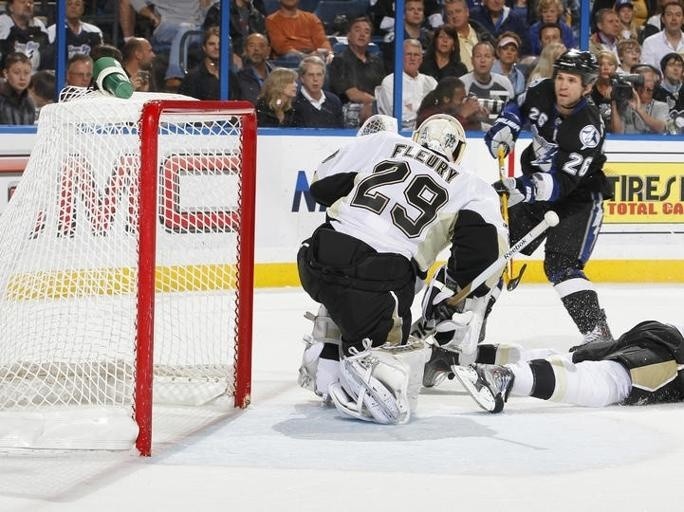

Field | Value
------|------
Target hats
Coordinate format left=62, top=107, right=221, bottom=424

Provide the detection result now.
left=615, top=0, right=633, bottom=10
left=497, top=37, right=519, bottom=48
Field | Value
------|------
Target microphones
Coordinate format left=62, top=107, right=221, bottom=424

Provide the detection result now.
left=647, top=88, right=657, bottom=91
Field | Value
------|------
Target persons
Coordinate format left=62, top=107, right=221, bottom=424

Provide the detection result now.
left=449, top=319, right=684, bottom=414
left=1, top=0, right=684, bottom=137
left=423, top=48, right=617, bottom=387
left=675, top=412, right=678, bottom=415
left=494, top=422, right=499, bottom=424
left=295, top=112, right=510, bottom=426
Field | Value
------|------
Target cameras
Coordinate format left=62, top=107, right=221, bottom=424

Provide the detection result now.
left=138, top=71, right=152, bottom=81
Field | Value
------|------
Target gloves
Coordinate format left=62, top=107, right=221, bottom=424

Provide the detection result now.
left=492, top=176, right=537, bottom=209
left=486, top=111, right=521, bottom=159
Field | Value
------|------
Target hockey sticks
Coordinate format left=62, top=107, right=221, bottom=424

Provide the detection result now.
left=496, top=146, right=526, bottom=291
left=412, top=209, right=562, bottom=340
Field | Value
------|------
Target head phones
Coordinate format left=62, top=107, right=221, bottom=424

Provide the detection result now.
left=630, top=64, right=661, bottom=89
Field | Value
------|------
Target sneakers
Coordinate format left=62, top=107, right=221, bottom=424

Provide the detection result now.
left=471, top=362, right=513, bottom=402
left=568, top=321, right=616, bottom=363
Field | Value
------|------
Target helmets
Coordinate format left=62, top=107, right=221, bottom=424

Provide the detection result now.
left=411, top=113, right=467, bottom=166
left=551, top=50, right=600, bottom=86
